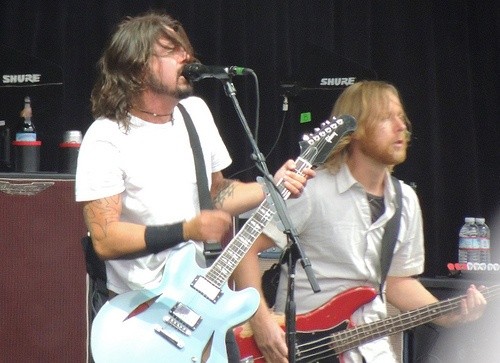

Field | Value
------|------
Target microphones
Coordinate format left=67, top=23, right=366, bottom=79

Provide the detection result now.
left=182, top=62, right=255, bottom=81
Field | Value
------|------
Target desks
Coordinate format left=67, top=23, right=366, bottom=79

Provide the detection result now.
left=404, top=274, right=500, bottom=363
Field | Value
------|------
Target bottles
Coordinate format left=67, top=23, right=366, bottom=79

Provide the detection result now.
left=16, top=96, right=36, bottom=142
left=475, top=218, right=490, bottom=272
left=459, top=217, right=479, bottom=274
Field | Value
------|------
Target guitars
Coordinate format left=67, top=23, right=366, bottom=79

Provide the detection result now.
left=89, top=113, right=358, bottom=363
left=232, top=280, right=500, bottom=363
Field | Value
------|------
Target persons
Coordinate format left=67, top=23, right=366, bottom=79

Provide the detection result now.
left=234, top=81, right=487, bottom=363
left=74, top=15, right=315, bottom=363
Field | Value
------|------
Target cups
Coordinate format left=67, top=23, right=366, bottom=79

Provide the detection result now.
left=64, top=130, right=82, bottom=143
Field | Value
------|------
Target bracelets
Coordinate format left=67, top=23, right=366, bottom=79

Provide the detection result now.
left=145, top=219, right=186, bottom=254
left=141, top=109, right=169, bottom=117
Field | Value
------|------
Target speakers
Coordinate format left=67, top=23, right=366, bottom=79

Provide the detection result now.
left=386, top=279, right=500, bottom=363
left=0, top=171, right=94, bottom=362
